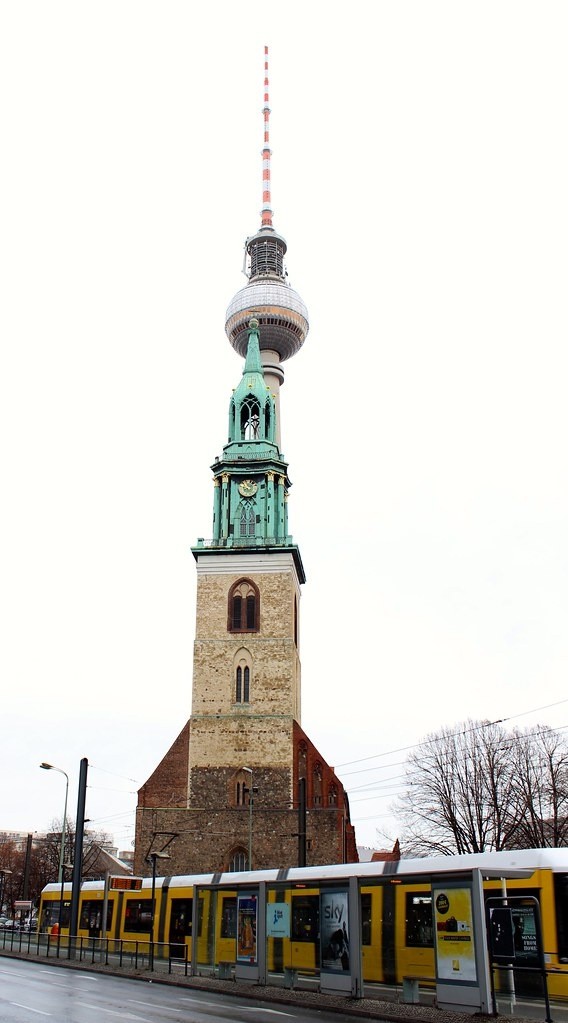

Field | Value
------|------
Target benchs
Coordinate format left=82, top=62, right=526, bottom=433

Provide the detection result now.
left=219, top=962, right=236, bottom=979
left=284, top=965, right=320, bottom=988
left=402, top=975, right=436, bottom=1004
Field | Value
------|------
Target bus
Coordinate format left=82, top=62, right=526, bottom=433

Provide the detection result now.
left=34, top=848, right=568, bottom=1006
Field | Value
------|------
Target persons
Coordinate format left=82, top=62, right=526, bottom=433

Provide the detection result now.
left=494, top=917, right=524, bottom=995
left=81, top=911, right=101, bottom=936
left=364, top=907, right=424, bottom=974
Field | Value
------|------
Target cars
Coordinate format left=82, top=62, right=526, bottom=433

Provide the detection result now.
left=0, top=916, right=38, bottom=932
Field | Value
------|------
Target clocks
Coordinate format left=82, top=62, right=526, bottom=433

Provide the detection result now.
left=238, top=479, right=257, bottom=497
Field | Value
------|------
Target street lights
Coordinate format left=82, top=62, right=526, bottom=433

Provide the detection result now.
left=0, top=869, right=13, bottom=923
left=147, top=852, right=172, bottom=971
left=242, top=766, right=253, bottom=870
left=57, top=862, right=73, bottom=959
left=39, top=763, right=69, bottom=883
